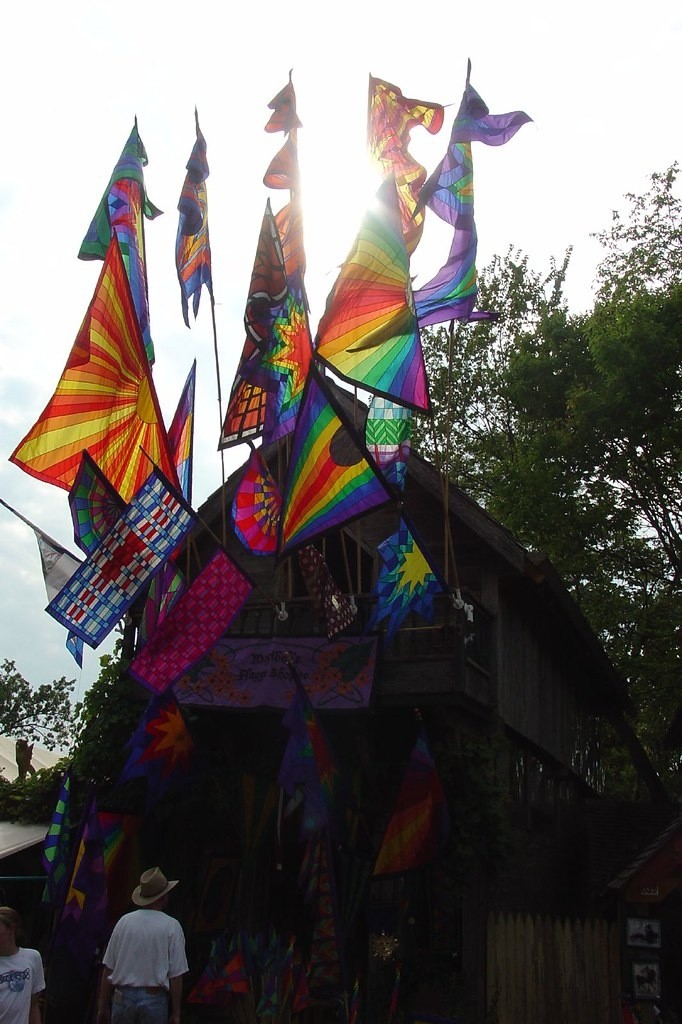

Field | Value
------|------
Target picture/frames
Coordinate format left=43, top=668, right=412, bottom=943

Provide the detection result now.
left=626, top=917, right=662, bottom=949
left=631, top=960, right=662, bottom=1001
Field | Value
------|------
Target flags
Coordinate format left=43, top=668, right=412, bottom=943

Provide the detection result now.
left=45, top=467, right=198, bottom=650
left=125, top=544, right=257, bottom=694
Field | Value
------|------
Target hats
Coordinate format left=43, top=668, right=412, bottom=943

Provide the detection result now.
left=131, top=867, right=180, bottom=906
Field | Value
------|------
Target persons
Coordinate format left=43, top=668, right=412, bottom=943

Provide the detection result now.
left=96, top=866, right=189, bottom=1024
left=0, top=906, right=46, bottom=1024
